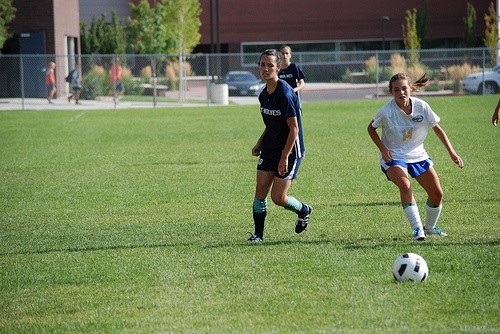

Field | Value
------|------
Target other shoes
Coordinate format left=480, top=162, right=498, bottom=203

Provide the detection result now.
left=75, top=102, right=81, bottom=104
left=68, top=96, right=71, bottom=102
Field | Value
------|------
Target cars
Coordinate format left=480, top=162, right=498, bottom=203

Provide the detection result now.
left=461, top=63, right=500, bottom=95
left=226, top=71, right=261, bottom=96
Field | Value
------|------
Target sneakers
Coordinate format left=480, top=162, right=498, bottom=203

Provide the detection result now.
left=246, top=232, right=263, bottom=243
left=412, top=227, right=426, bottom=241
left=423, top=226, right=447, bottom=237
left=295, top=204, right=312, bottom=233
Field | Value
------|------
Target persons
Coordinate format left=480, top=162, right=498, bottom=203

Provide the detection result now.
left=278, top=43, right=305, bottom=159
left=367, top=72, right=464, bottom=241
left=492, top=99, right=500, bottom=126
left=42, top=61, right=124, bottom=105
left=243, top=48, right=313, bottom=243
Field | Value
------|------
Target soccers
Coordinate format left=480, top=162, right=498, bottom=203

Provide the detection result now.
left=393, top=253, right=428, bottom=283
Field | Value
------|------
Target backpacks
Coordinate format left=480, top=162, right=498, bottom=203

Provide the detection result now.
left=65, top=69, right=79, bottom=82
